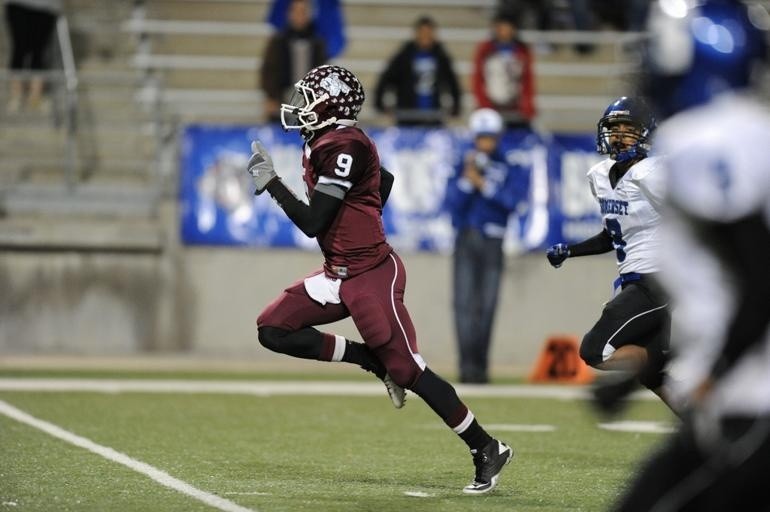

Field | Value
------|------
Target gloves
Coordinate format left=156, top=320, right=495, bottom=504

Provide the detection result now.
left=547, top=243, right=570, bottom=268
left=247, top=141, right=279, bottom=195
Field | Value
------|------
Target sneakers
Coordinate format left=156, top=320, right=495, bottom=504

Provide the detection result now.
left=463, top=438, right=514, bottom=493
left=384, top=373, right=406, bottom=408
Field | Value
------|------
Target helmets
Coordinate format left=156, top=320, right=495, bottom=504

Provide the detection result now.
left=596, top=96, right=658, bottom=161
left=280, top=65, right=365, bottom=133
left=469, top=108, right=500, bottom=135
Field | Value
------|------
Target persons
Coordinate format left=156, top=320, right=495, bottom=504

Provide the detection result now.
left=471, top=10, right=534, bottom=121
left=442, top=107, right=531, bottom=385
left=268, top=0, right=347, bottom=60
left=545, top=96, right=711, bottom=428
left=247, top=64, right=513, bottom=495
left=375, top=19, right=456, bottom=134
left=613, top=0, right=769, bottom=512
